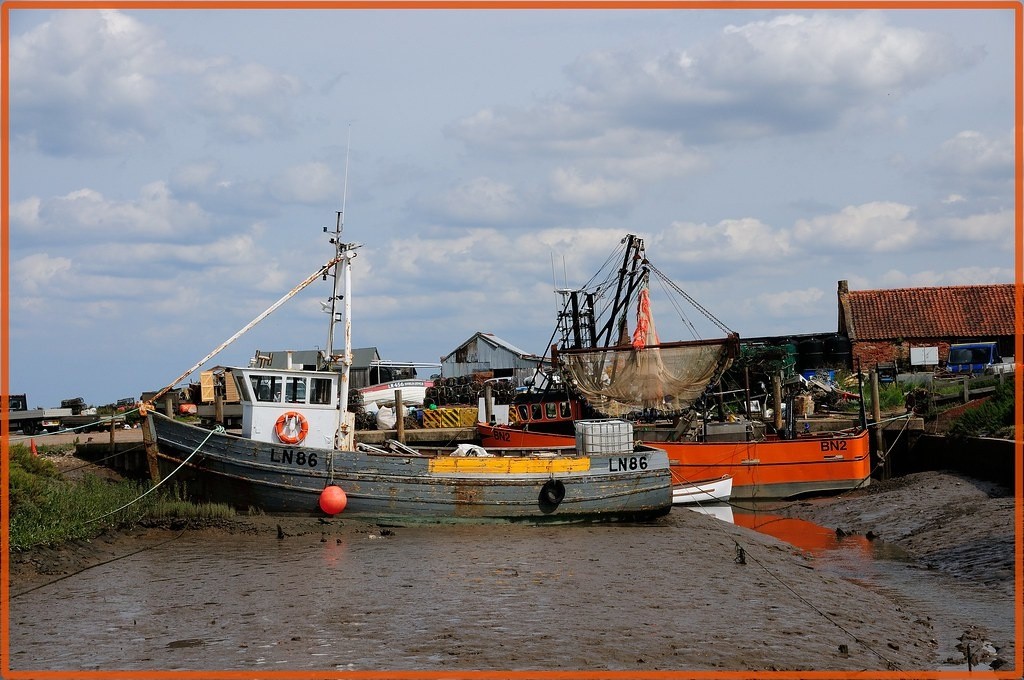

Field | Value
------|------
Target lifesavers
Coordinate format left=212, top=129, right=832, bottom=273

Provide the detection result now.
left=543, top=480, right=566, bottom=502
left=271, top=410, right=311, bottom=447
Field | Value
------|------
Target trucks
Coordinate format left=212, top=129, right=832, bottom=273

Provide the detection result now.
left=9, top=393, right=73, bottom=436
left=37, top=407, right=127, bottom=433
left=945, top=342, right=1004, bottom=379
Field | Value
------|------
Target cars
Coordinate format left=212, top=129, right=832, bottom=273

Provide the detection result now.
left=123, top=424, right=133, bottom=430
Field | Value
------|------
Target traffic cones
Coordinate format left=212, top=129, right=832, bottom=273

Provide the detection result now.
left=29, top=438, right=39, bottom=459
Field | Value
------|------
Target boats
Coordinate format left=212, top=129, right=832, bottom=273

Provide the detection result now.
left=335, top=378, right=435, bottom=409
left=475, top=230, right=871, bottom=500
left=671, top=476, right=735, bottom=505
left=142, top=210, right=675, bottom=523
